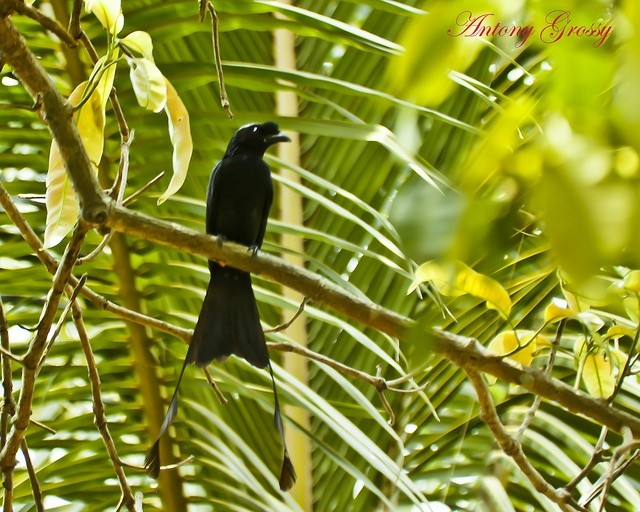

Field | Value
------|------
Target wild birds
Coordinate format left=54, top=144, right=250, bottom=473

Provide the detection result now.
left=144, top=120, right=297, bottom=492
